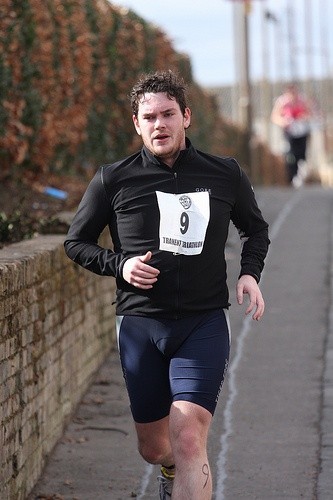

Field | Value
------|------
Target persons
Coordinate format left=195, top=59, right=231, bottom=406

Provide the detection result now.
left=274, top=78, right=313, bottom=189
left=63, top=70, right=272, bottom=500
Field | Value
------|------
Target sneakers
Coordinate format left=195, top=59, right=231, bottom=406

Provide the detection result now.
left=157, top=467, right=175, bottom=500
